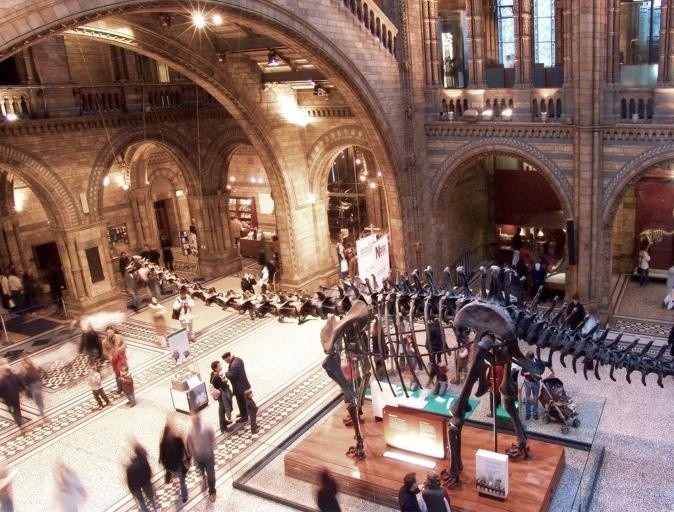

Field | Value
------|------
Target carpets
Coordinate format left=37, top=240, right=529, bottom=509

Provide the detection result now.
left=0, top=311, right=63, bottom=340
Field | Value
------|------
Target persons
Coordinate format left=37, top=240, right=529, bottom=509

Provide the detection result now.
left=421, top=470, right=451, bottom=512
left=317, top=470, right=341, bottom=512
left=335, top=233, right=358, bottom=278
left=662, top=265, right=674, bottom=311
left=511, top=227, right=522, bottom=268
left=398, top=472, right=428, bottom=512
left=566, top=295, right=584, bottom=340
left=344, top=315, right=576, bottom=421
left=229, top=215, right=244, bottom=246
left=638, top=243, right=651, bottom=286
left=581, top=304, right=599, bottom=340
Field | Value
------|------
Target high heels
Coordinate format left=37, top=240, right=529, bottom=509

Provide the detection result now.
left=221, top=428, right=233, bottom=434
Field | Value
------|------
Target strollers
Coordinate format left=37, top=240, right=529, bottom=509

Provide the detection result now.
left=536, top=376, right=581, bottom=435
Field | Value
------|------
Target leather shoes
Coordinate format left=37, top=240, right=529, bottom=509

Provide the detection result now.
left=236, top=415, right=248, bottom=422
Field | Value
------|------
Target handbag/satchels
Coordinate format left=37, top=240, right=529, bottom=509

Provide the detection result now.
left=171, top=299, right=182, bottom=320
left=209, top=385, right=220, bottom=400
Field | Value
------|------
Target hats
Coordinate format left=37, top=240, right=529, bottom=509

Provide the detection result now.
left=427, top=469, right=440, bottom=481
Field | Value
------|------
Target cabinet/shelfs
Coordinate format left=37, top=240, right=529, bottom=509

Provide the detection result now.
left=226, top=194, right=258, bottom=234
left=240, top=236, right=274, bottom=260
